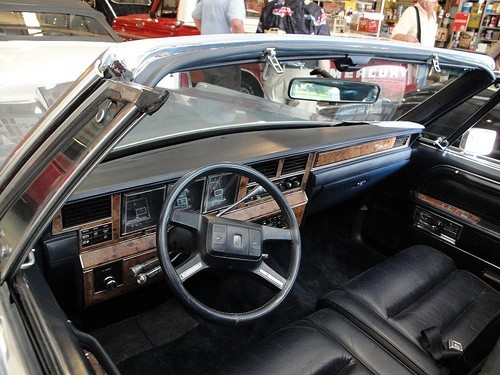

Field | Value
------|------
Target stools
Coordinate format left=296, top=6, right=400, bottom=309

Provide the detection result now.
left=224, top=244, right=500, bottom=375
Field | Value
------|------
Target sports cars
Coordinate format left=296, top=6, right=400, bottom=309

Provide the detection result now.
left=0, top=26, right=500, bottom=375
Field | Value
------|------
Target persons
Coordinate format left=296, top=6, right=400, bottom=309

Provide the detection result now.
left=331, top=10, right=353, bottom=25
left=391, top=0, right=439, bottom=87
left=254, top=0, right=331, bottom=113
left=192, top=0, right=246, bottom=90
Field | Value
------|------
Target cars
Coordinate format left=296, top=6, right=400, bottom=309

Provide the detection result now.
left=0, top=0, right=417, bottom=107
